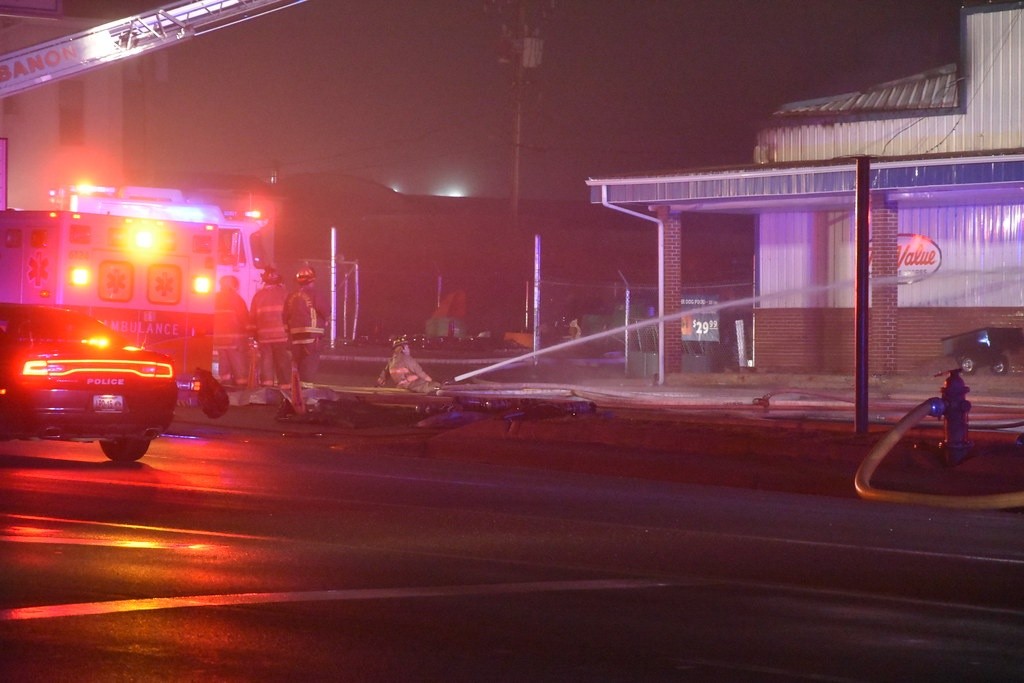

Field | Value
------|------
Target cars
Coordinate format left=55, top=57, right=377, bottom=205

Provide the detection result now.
left=0, top=301, right=178, bottom=462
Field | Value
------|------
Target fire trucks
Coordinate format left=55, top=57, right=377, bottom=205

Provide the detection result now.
left=72, top=198, right=268, bottom=314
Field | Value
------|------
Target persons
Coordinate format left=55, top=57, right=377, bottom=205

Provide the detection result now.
left=214, top=275, right=254, bottom=387
left=245, top=269, right=293, bottom=386
left=283, top=265, right=328, bottom=383
left=376, top=337, right=443, bottom=393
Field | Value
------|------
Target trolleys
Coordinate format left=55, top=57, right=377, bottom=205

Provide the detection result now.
left=941, top=326, right=1024, bottom=376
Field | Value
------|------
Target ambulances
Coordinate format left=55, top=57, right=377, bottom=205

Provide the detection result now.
left=0, top=208, right=219, bottom=412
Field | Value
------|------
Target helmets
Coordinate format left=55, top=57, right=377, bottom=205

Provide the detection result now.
left=391, top=337, right=408, bottom=351
left=293, top=266, right=316, bottom=286
